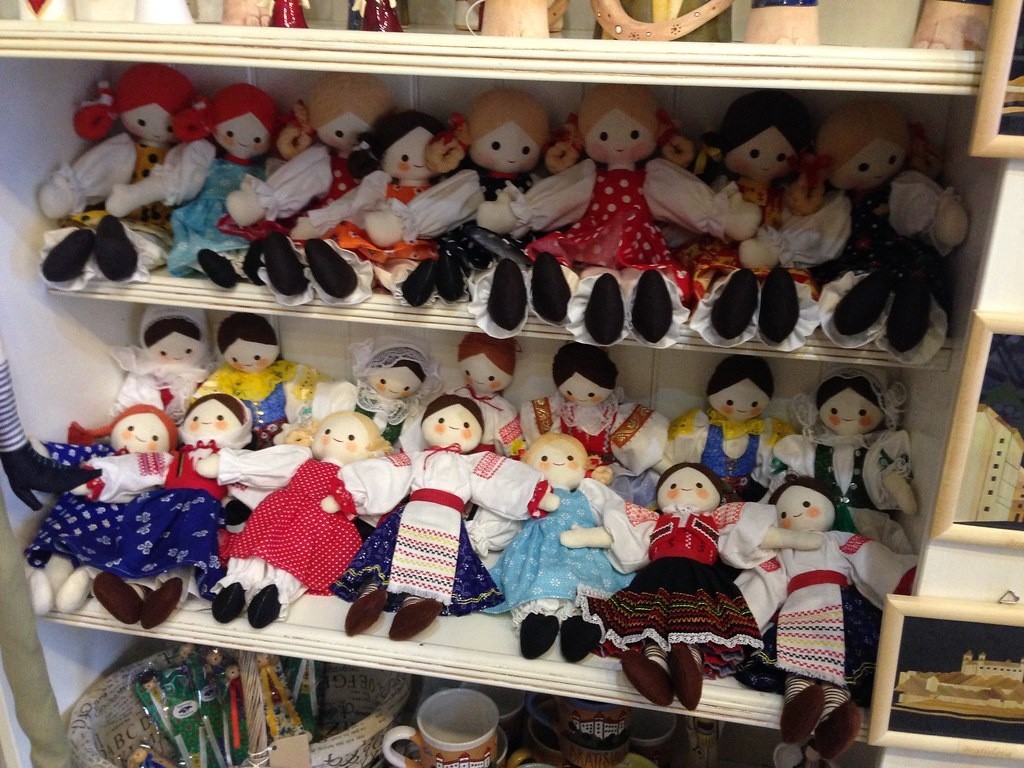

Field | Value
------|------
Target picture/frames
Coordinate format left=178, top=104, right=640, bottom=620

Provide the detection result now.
left=864, top=590, right=1024, bottom=758
left=968, top=0, right=1024, bottom=158
left=927, top=309, right=1024, bottom=550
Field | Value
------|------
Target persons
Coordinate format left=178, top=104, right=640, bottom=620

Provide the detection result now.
left=40, top=64, right=969, bottom=368
left=259, top=0, right=311, bottom=28
left=24, top=302, right=918, bottom=758
left=0, top=340, right=101, bottom=511
left=352, top=0, right=403, bottom=32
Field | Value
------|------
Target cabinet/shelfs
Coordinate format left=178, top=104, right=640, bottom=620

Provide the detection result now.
left=0, top=1, right=984, bottom=768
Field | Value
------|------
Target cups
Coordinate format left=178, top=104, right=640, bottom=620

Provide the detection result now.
left=382, top=676, right=680, bottom=768
